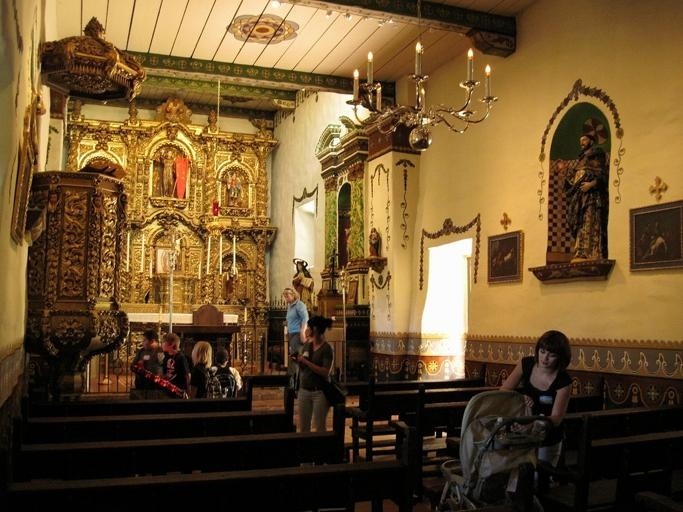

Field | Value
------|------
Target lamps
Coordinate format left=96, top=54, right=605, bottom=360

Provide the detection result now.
left=346, top=1, right=498, bottom=150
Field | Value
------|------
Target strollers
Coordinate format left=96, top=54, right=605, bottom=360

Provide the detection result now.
left=429, top=387, right=561, bottom=509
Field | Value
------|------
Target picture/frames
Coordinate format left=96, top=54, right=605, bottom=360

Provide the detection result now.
left=630, top=199, right=682, bottom=272
left=488, top=230, right=523, bottom=284
left=10, top=138, right=34, bottom=245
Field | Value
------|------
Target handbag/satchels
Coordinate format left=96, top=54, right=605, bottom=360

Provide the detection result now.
left=321, top=379, right=348, bottom=406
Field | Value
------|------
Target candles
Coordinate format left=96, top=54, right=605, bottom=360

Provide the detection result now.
left=207, top=233, right=236, bottom=276
left=127, top=232, right=153, bottom=278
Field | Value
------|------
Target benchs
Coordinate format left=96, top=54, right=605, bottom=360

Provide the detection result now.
left=5, top=396, right=400, bottom=510
left=336, top=379, right=502, bottom=510
left=536, top=394, right=681, bottom=512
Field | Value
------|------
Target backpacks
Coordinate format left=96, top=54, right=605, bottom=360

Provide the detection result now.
left=194, top=366, right=238, bottom=399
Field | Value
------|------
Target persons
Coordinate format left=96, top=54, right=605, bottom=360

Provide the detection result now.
left=566, top=118, right=611, bottom=261
left=130, top=330, right=164, bottom=389
left=187, top=340, right=213, bottom=399
left=211, top=347, right=243, bottom=399
left=499, top=329, right=572, bottom=480
left=160, top=148, right=177, bottom=197
left=226, top=169, right=243, bottom=207
left=292, top=261, right=315, bottom=313
left=283, top=287, right=310, bottom=389
left=158, top=331, right=187, bottom=399
left=294, top=314, right=334, bottom=433
